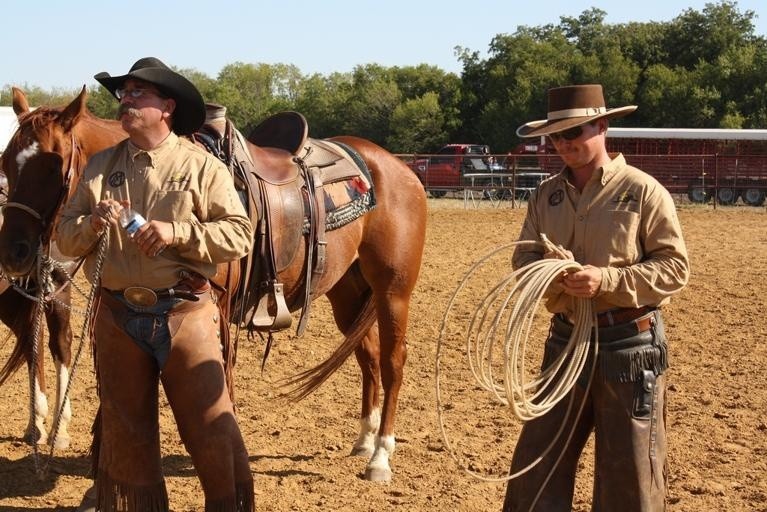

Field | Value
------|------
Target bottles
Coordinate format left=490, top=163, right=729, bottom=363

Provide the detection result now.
left=117, top=205, right=165, bottom=258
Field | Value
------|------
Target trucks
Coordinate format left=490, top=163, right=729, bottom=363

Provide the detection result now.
left=502, top=133, right=765, bottom=209
left=408, top=143, right=539, bottom=201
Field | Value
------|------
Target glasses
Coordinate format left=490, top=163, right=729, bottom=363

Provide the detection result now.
left=550, top=126, right=582, bottom=141
left=115, top=87, right=145, bottom=99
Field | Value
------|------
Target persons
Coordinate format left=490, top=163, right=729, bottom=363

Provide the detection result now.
left=500, top=81, right=693, bottom=510
left=48, top=55, right=257, bottom=510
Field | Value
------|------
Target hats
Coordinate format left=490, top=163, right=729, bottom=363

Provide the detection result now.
left=94, top=57, right=206, bottom=136
left=516, top=83, right=638, bottom=137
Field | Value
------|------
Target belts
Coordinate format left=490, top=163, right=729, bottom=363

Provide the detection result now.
left=556, top=306, right=656, bottom=336
left=103, top=289, right=201, bottom=303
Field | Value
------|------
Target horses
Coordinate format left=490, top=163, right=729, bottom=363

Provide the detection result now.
left=0, top=86, right=431, bottom=482
left=0, top=169, right=79, bottom=453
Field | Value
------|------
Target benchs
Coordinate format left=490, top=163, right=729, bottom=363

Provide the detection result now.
left=462, top=173, right=550, bottom=208
